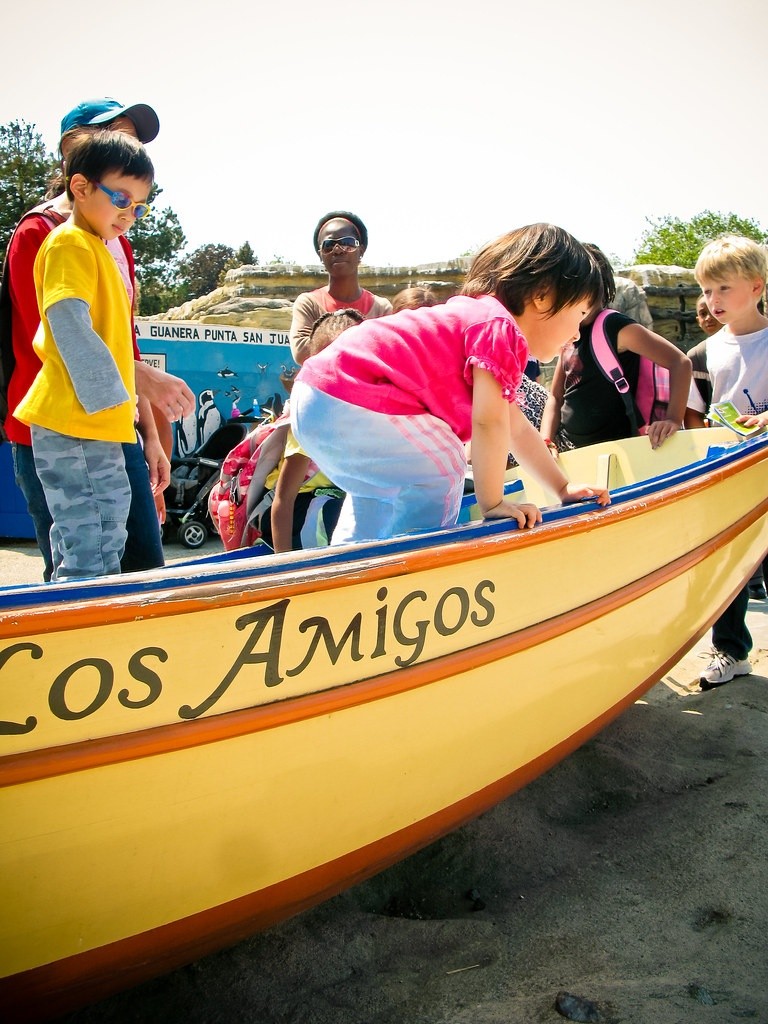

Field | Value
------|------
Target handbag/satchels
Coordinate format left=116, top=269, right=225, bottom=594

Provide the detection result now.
left=589, top=308, right=683, bottom=436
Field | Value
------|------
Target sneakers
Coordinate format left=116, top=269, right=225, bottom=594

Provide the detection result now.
left=697, top=646, right=753, bottom=690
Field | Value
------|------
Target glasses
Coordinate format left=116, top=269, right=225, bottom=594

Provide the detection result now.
left=66, top=172, right=151, bottom=219
left=318, top=236, right=359, bottom=256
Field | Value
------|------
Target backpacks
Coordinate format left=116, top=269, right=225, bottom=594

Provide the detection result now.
left=208, top=418, right=292, bottom=552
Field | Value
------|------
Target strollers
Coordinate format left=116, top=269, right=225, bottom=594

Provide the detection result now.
left=161, top=407, right=275, bottom=549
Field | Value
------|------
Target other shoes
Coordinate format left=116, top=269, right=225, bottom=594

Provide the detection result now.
left=747, top=584, right=767, bottom=599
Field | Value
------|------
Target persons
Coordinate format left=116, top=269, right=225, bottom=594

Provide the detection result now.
left=687, top=236, right=768, bottom=688
left=3, top=96, right=196, bottom=582
left=254, top=211, right=692, bottom=553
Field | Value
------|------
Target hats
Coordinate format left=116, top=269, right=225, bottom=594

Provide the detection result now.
left=313, top=211, right=368, bottom=253
left=61, top=98, right=160, bottom=144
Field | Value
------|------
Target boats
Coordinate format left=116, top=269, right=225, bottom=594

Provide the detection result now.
left=0, top=428, right=767, bottom=1024
